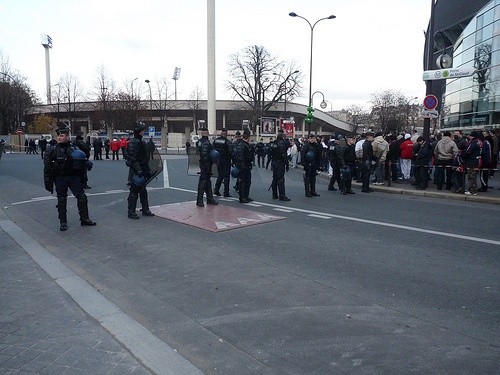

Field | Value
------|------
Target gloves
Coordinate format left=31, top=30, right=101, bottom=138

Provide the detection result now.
left=135, top=169, right=143, bottom=177
left=286, top=166, right=289, bottom=172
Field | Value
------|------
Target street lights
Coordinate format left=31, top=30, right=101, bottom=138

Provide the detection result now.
left=272, top=71, right=301, bottom=142
left=399, top=97, right=418, bottom=129
left=288, top=12, right=336, bottom=140
left=144, top=80, right=152, bottom=142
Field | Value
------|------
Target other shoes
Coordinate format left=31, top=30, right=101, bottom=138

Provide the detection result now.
left=214, top=191, right=221, bottom=196
left=341, top=189, right=355, bottom=195
left=465, top=191, right=477, bottom=196
left=196, top=202, right=204, bottom=207
left=306, top=191, right=320, bottom=198
left=233, top=186, right=238, bottom=191
left=128, top=214, right=139, bottom=219
left=273, top=194, right=291, bottom=201
left=361, top=188, right=374, bottom=193
left=373, top=182, right=384, bottom=186
left=207, top=200, right=218, bottom=205
left=328, top=187, right=337, bottom=191
left=60, top=223, right=68, bottom=231
left=224, top=195, right=232, bottom=197
left=240, top=198, right=254, bottom=203
left=142, top=211, right=155, bottom=216
left=81, top=220, right=96, bottom=226
left=478, top=187, right=488, bottom=192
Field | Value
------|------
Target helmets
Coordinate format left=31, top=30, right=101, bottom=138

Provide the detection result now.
left=210, top=150, right=220, bottom=162
left=71, top=150, right=86, bottom=160
left=231, top=167, right=239, bottom=178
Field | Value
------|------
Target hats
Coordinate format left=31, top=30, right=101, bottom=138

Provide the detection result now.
left=132, top=124, right=147, bottom=133
left=56, top=128, right=71, bottom=135
left=405, top=134, right=411, bottom=140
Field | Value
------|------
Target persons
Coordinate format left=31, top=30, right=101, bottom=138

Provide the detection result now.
left=186, top=140, right=191, bottom=155
left=24, top=136, right=57, bottom=159
left=0, top=139, right=6, bottom=159
left=124, top=123, right=155, bottom=219
left=75, top=132, right=129, bottom=161
left=42, top=127, right=97, bottom=231
left=195, top=124, right=500, bottom=207
